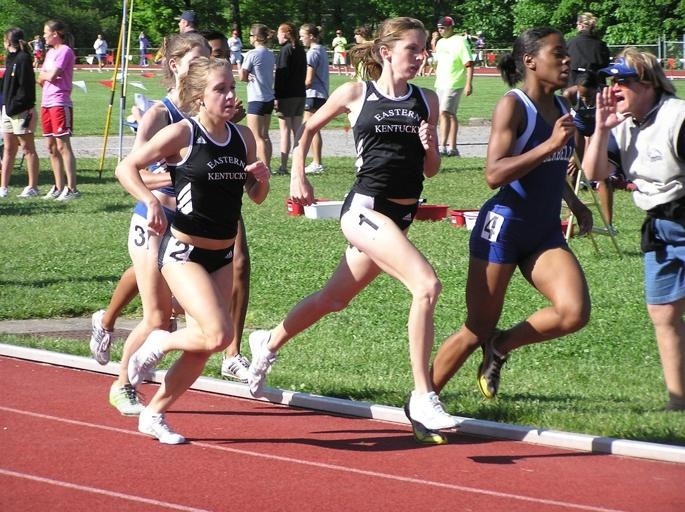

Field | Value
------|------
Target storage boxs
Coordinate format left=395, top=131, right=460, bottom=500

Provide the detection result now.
left=415, top=204, right=482, bottom=232
left=561, top=219, right=577, bottom=238
left=303, top=200, right=345, bottom=219
left=285, top=197, right=328, bottom=216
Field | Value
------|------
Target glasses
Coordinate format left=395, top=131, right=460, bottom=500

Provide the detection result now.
left=610, top=78, right=639, bottom=85
left=249, top=34, right=254, bottom=37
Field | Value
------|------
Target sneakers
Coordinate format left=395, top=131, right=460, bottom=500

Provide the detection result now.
left=404, top=403, right=448, bottom=444
left=220, top=353, right=251, bottom=380
left=55, top=186, right=81, bottom=201
left=304, top=163, right=324, bottom=174
left=108, top=380, right=146, bottom=417
left=90, top=309, right=114, bottom=365
left=128, top=329, right=170, bottom=387
left=447, top=148, right=459, bottom=157
left=16, top=186, right=39, bottom=197
left=247, top=330, right=276, bottom=397
left=409, top=395, right=461, bottom=430
left=0, top=186, right=8, bottom=196
left=273, top=167, right=288, bottom=175
left=138, top=409, right=187, bottom=444
left=477, top=328, right=511, bottom=399
left=40, top=185, right=63, bottom=199
left=438, top=146, right=447, bottom=154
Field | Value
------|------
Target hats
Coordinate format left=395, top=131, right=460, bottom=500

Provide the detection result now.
left=174, top=10, right=195, bottom=24
left=437, top=16, right=455, bottom=28
left=598, top=57, right=658, bottom=80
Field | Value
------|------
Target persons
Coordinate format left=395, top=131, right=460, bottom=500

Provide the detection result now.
left=404, top=25, right=593, bottom=447
left=582, top=45, right=684, bottom=414
left=247, top=14, right=460, bottom=432
left=89, top=31, right=252, bottom=383
left=109, top=34, right=212, bottom=416
left=88, top=57, right=271, bottom=447
left=1, top=10, right=684, bottom=203
